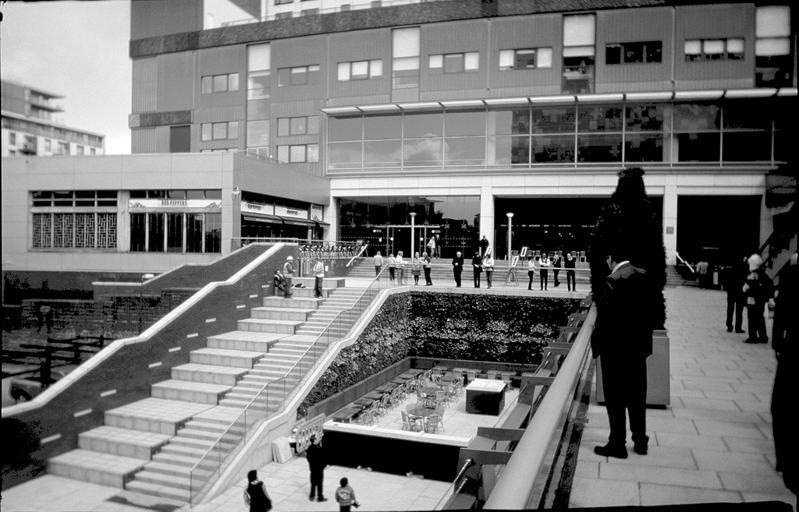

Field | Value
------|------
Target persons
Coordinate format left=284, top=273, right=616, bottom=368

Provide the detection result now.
left=412, top=251, right=423, bottom=286
left=590, top=240, right=656, bottom=460
left=451, top=251, right=464, bottom=288
left=372, top=250, right=383, bottom=280
left=427, top=232, right=441, bottom=258
left=539, top=252, right=550, bottom=291
left=420, top=252, right=433, bottom=286
left=471, top=234, right=495, bottom=289
left=551, top=254, right=561, bottom=287
left=564, top=252, right=576, bottom=291
left=243, top=469, right=273, bottom=512
left=312, top=257, right=325, bottom=299
left=387, top=253, right=396, bottom=280
left=283, top=256, right=296, bottom=299
left=394, top=250, right=408, bottom=287
left=305, top=435, right=329, bottom=502
left=335, top=476, right=361, bottom=512
left=273, top=269, right=294, bottom=292
left=697, top=252, right=799, bottom=352
left=527, top=256, right=536, bottom=290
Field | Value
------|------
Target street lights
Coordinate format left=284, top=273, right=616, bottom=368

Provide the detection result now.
left=505, top=211, right=515, bottom=267
left=36, top=303, right=55, bottom=392
left=409, top=212, right=418, bottom=263
left=139, top=272, right=157, bottom=335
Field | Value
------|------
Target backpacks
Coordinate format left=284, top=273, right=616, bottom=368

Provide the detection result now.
left=752, top=272, right=776, bottom=304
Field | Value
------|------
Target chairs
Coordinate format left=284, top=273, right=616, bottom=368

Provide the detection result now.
left=330, top=365, right=521, bottom=434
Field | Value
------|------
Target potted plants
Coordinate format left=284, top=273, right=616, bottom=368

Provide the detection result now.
left=593, top=166, right=671, bottom=409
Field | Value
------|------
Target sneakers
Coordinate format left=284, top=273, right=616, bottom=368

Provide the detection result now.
left=727, top=327, right=767, bottom=344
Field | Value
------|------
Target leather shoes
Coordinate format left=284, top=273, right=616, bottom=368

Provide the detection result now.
left=527, top=282, right=576, bottom=292
left=317, top=498, right=327, bottom=501
left=633, top=444, right=648, bottom=455
left=398, top=282, right=492, bottom=289
left=594, top=443, right=628, bottom=459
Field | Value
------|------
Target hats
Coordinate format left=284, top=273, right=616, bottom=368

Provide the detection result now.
left=748, top=255, right=764, bottom=270
left=287, top=255, right=295, bottom=260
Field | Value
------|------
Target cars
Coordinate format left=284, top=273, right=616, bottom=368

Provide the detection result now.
left=7, top=364, right=81, bottom=407
